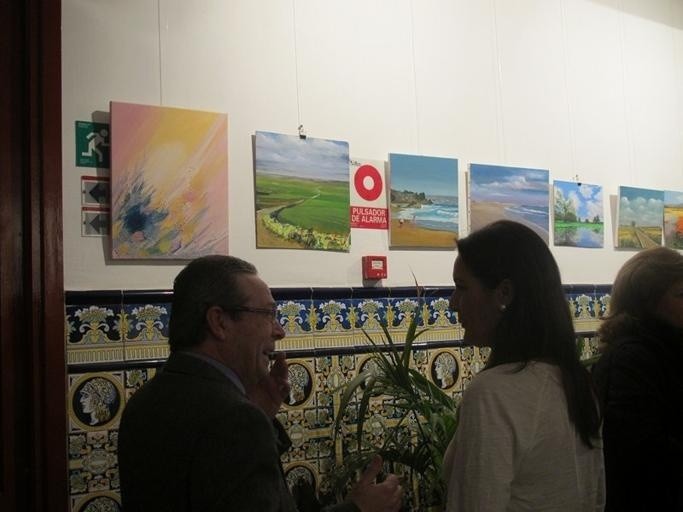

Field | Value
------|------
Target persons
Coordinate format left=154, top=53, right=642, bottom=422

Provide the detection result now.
left=118, top=255, right=404, bottom=512
left=442, top=221, right=607, bottom=512
left=591, top=248, right=683, bottom=511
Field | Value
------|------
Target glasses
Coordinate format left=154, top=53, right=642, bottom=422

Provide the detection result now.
left=224, top=304, right=276, bottom=323
